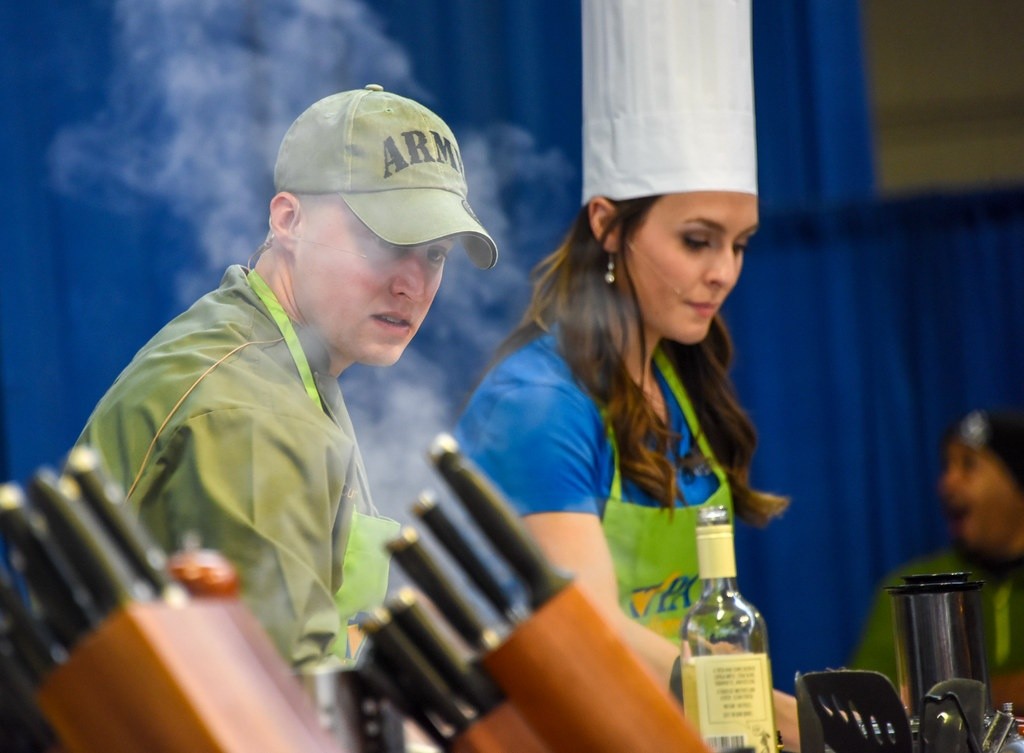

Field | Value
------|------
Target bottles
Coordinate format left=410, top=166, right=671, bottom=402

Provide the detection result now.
left=680, top=505, right=778, bottom=752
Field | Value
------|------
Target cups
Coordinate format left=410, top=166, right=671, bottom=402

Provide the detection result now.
left=887, top=571, right=992, bottom=726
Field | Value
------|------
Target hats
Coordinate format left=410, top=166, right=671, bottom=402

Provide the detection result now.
left=946, top=409, right=1024, bottom=488
left=580, top=0, right=757, bottom=208
left=274, top=85, right=499, bottom=268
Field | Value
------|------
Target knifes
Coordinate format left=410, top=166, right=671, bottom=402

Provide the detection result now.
left=0, top=443, right=190, bottom=696
left=357, top=434, right=574, bottom=751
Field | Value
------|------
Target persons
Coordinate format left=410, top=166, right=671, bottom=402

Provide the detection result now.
left=30, top=84, right=498, bottom=753
left=454, top=0, right=801, bottom=753
left=848, top=408, right=1024, bottom=723
